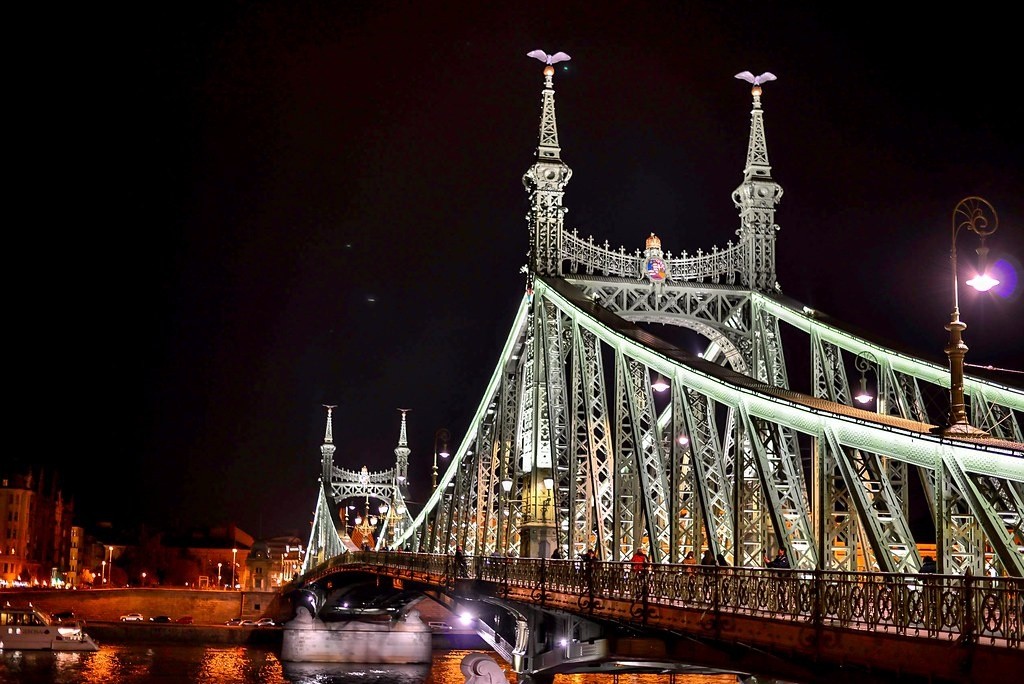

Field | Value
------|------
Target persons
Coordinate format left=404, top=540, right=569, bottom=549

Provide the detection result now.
left=455, top=545, right=469, bottom=578
left=717, top=554, right=731, bottom=598
left=629, top=549, right=649, bottom=573
left=490, top=549, right=500, bottom=557
left=551, top=549, right=561, bottom=559
left=360, top=543, right=425, bottom=563
left=917, top=556, right=936, bottom=592
left=681, top=551, right=697, bottom=591
left=577, top=549, right=599, bottom=560
left=506, top=550, right=514, bottom=558
left=763, top=546, right=791, bottom=578
left=701, top=550, right=715, bottom=573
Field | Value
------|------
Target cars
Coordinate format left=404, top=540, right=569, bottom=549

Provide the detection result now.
left=55, top=612, right=75, bottom=619
left=148, top=616, right=171, bottom=623
left=175, top=616, right=194, bottom=624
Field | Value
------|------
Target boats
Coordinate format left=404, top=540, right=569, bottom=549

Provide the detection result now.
left=0, top=607, right=99, bottom=651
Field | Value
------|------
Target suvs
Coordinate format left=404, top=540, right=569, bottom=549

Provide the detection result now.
left=120, top=613, right=143, bottom=622
left=224, top=618, right=285, bottom=627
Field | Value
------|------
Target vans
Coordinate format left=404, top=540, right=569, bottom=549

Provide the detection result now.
left=428, top=622, right=452, bottom=631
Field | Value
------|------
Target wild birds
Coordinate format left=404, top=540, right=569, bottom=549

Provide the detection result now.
left=734, top=70, right=778, bottom=85
left=527, top=49, right=572, bottom=66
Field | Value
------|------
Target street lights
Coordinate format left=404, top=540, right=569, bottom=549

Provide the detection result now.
left=855, top=351, right=881, bottom=415
left=939, top=197, right=1000, bottom=439
left=108, top=546, right=113, bottom=584
left=232, top=547, right=237, bottom=591
left=218, top=563, right=222, bottom=589
left=102, top=560, right=106, bottom=585
left=430, top=428, right=452, bottom=496
